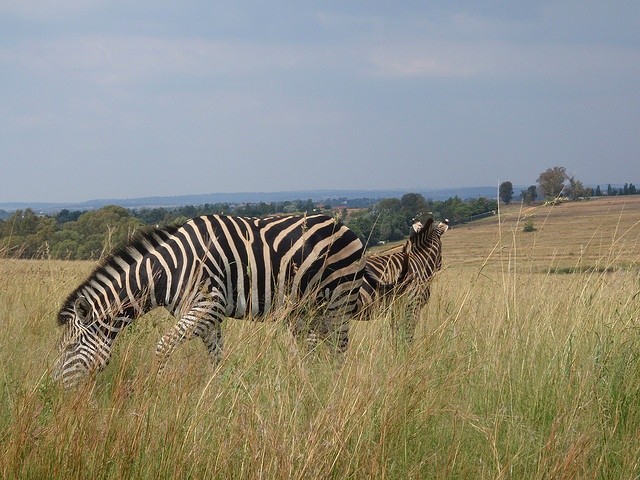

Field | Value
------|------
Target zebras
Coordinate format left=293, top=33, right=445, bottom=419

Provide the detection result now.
left=56, top=213, right=366, bottom=396
left=351, top=218, right=451, bottom=345
